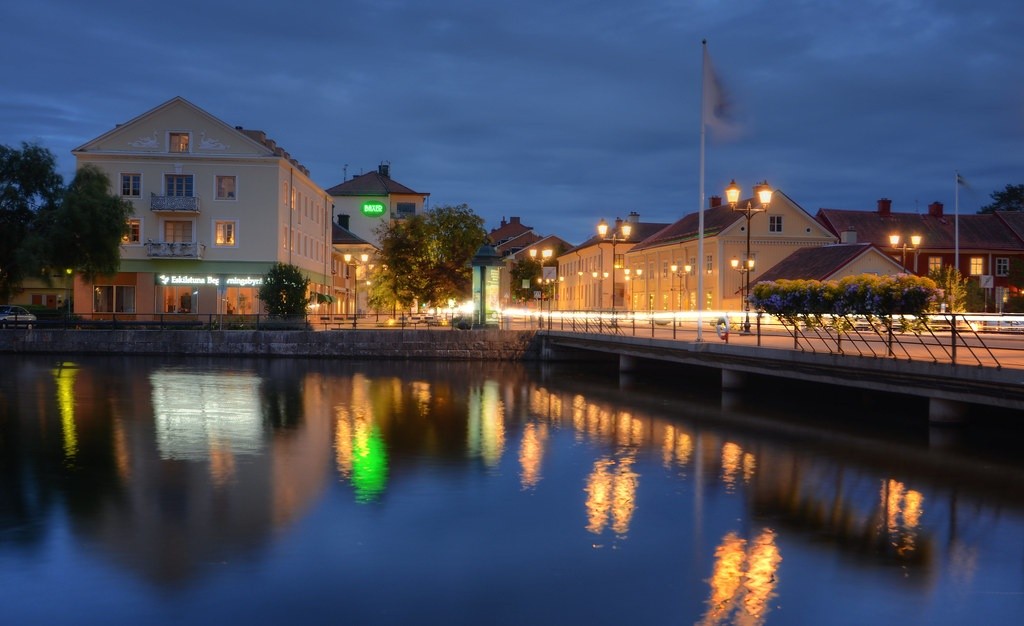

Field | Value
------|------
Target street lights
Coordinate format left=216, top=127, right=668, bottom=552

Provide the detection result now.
left=730, top=255, right=755, bottom=336
left=671, top=261, right=691, bottom=327
left=624, top=266, right=643, bottom=324
left=344, top=253, right=369, bottom=331
left=888, top=231, right=923, bottom=274
left=537, top=278, right=549, bottom=312
left=593, top=271, right=609, bottom=307
left=725, top=178, right=774, bottom=336
left=550, top=276, right=565, bottom=311
left=597, top=218, right=632, bottom=329
left=530, top=245, right=553, bottom=329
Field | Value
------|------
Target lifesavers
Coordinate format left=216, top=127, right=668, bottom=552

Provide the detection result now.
left=716, top=316, right=730, bottom=340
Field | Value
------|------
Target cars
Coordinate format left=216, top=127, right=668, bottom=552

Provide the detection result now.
left=0, top=305, right=37, bottom=330
left=66, top=266, right=73, bottom=319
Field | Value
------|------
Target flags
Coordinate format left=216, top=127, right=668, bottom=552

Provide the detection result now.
left=957, top=173, right=967, bottom=184
left=702, top=44, right=748, bottom=134
